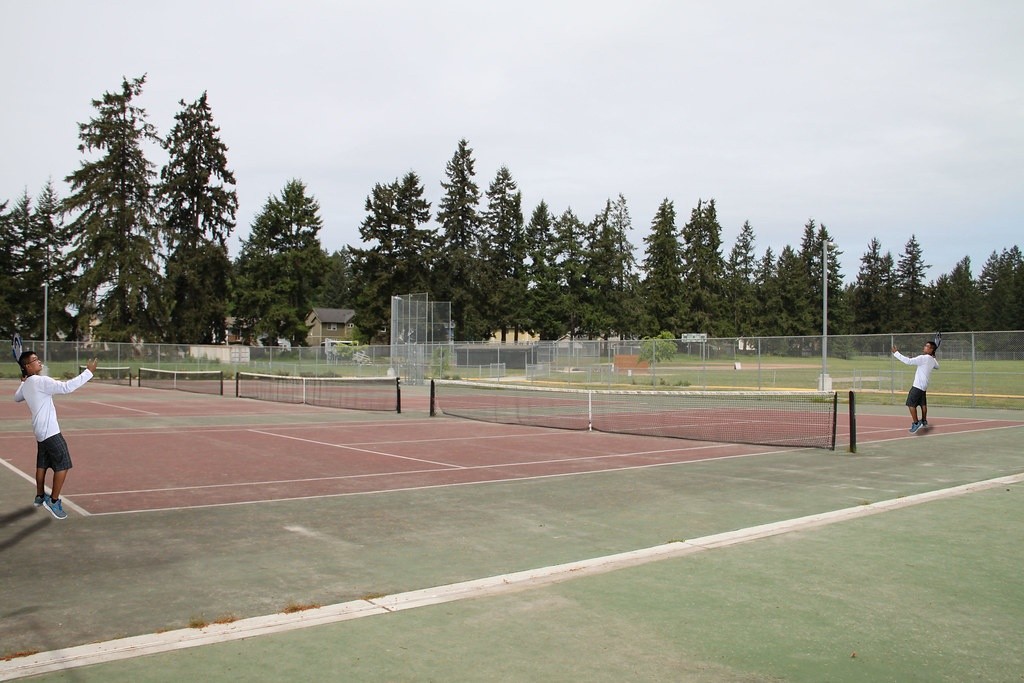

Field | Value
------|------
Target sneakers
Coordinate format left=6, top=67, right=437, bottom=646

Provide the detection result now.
left=918, top=419, right=927, bottom=425
left=34, top=493, right=48, bottom=507
left=43, top=498, right=68, bottom=519
left=910, top=422, right=923, bottom=433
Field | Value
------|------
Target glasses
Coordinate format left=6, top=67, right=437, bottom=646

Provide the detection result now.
left=25, top=357, right=40, bottom=367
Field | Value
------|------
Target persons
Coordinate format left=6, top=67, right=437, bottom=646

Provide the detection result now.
left=13, top=351, right=98, bottom=519
left=891, top=341, right=939, bottom=433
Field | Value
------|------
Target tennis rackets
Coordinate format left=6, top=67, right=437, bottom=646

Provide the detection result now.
left=12, top=331, right=26, bottom=378
left=932, top=328, right=942, bottom=358
